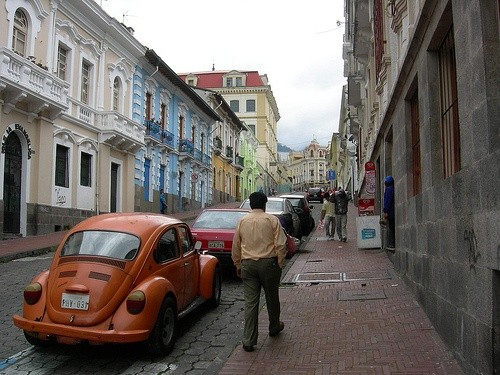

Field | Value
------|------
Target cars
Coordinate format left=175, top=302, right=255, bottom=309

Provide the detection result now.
left=238, top=196, right=304, bottom=243
left=13, top=212, right=224, bottom=357
left=319, top=188, right=327, bottom=198
left=279, top=194, right=315, bottom=235
left=191, top=208, right=251, bottom=269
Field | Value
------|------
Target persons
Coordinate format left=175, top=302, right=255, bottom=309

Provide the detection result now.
left=319, top=188, right=352, bottom=242
left=271, top=189, right=275, bottom=197
left=231, top=192, right=287, bottom=351
left=382, top=176, right=395, bottom=247
left=159, top=189, right=168, bottom=214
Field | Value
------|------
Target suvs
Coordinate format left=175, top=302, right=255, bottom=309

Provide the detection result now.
left=305, top=188, right=324, bottom=203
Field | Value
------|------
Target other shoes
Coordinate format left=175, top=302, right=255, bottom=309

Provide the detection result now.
left=243, top=344, right=254, bottom=352
left=269, top=321, right=285, bottom=336
left=327, top=236, right=334, bottom=240
left=339, top=237, right=346, bottom=242
left=388, top=243, right=395, bottom=248
